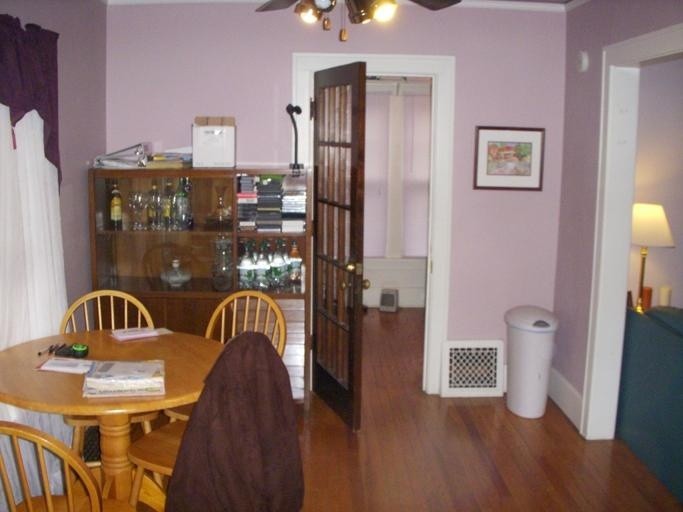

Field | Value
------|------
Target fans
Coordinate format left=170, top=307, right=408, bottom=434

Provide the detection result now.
left=251, top=0, right=466, bottom=16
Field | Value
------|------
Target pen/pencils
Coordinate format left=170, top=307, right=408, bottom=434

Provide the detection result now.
left=38, top=344, right=75, bottom=355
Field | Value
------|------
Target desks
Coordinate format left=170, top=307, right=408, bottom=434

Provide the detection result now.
left=1, top=328, right=225, bottom=509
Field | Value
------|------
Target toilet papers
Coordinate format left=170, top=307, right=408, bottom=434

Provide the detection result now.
left=659, top=286, right=672, bottom=307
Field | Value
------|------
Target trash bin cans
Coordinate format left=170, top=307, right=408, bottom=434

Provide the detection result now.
left=504, top=305, right=560, bottom=419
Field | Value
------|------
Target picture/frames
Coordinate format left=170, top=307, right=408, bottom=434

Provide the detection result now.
left=472, top=124, right=547, bottom=194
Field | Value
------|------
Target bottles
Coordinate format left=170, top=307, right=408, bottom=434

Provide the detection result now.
left=206, top=186, right=233, bottom=225
left=149, top=177, right=190, bottom=230
left=107, top=177, right=122, bottom=229
left=239, top=238, right=302, bottom=289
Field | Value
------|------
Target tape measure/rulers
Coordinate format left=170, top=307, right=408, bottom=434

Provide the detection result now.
left=72, top=343, right=88, bottom=358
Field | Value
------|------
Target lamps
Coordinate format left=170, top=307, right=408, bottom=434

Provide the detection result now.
left=296, top=0, right=398, bottom=43
left=626, top=201, right=678, bottom=312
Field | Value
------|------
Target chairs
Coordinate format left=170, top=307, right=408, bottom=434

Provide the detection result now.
left=132, top=333, right=301, bottom=512
left=166, top=289, right=288, bottom=422
left=0, top=420, right=135, bottom=512
left=58, top=290, right=161, bottom=456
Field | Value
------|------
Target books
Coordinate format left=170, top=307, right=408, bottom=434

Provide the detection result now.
left=91, top=152, right=194, bottom=171
left=236, top=173, right=306, bottom=234
left=82, top=358, right=165, bottom=400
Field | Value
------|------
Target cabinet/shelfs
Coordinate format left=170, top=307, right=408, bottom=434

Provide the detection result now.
left=85, top=161, right=320, bottom=412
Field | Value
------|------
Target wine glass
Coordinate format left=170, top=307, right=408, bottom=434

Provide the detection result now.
left=127, top=189, right=150, bottom=231
